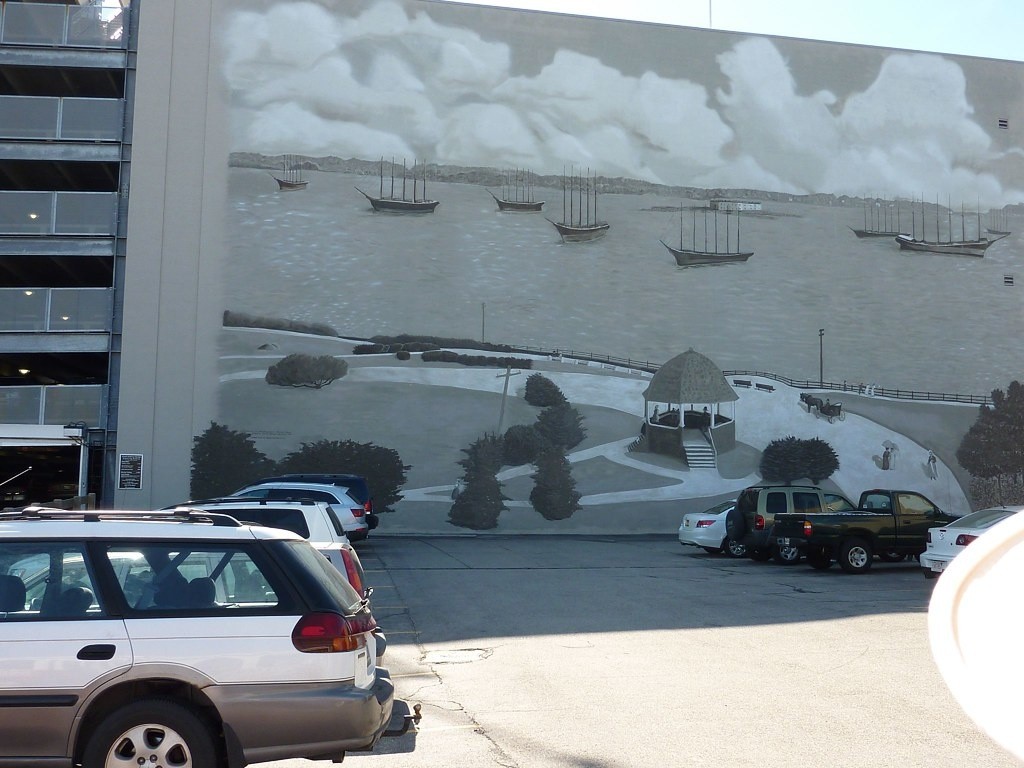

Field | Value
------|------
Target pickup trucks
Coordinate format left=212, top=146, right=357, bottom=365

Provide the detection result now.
left=774, top=489, right=951, bottom=576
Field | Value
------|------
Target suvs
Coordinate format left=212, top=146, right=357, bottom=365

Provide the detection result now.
left=1, top=504, right=422, bottom=768
left=1, top=472, right=383, bottom=621
left=725, top=483, right=858, bottom=565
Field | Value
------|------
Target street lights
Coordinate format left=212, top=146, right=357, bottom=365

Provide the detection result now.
left=819, top=328, right=825, bottom=389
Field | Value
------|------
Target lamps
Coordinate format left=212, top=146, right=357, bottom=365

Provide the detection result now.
left=63, top=421, right=91, bottom=448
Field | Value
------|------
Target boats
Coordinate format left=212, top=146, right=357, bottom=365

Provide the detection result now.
left=658, top=202, right=756, bottom=267
left=543, top=164, right=611, bottom=246
left=352, top=155, right=441, bottom=213
left=846, top=202, right=912, bottom=239
left=484, top=167, right=547, bottom=214
left=988, top=208, right=1011, bottom=235
left=896, top=193, right=1007, bottom=258
left=269, top=154, right=311, bottom=192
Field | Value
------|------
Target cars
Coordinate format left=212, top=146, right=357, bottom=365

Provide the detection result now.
left=679, top=498, right=751, bottom=558
left=920, top=503, right=1023, bottom=581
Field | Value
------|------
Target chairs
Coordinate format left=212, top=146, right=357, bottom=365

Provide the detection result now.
left=0, top=575, right=26, bottom=618
left=865, top=501, right=872, bottom=508
left=883, top=502, right=891, bottom=509
left=150, top=573, right=217, bottom=611
left=45, top=588, right=93, bottom=615
